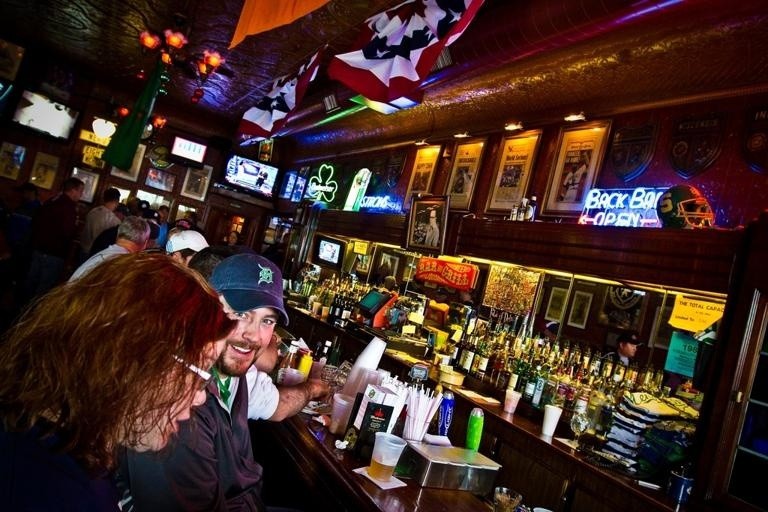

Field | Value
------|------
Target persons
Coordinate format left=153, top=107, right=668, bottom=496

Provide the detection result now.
left=256, top=168, right=267, bottom=186
left=608, top=329, right=647, bottom=366
left=351, top=257, right=477, bottom=307
left=425, top=210, right=439, bottom=247
left=545, top=322, right=560, bottom=340
left=562, top=151, right=589, bottom=202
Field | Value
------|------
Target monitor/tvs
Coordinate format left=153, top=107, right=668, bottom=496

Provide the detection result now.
left=356, top=288, right=392, bottom=315
left=215, top=142, right=284, bottom=202
left=289, top=176, right=307, bottom=203
left=280, top=172, right=297, bottom=199
left=168, top=132, right=210, bottom=169
left=312, top=235, right=346, bottom=271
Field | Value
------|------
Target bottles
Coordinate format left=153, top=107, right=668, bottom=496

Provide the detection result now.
left=277, top=342, right=312, bottom=386
left=449, top=309, right=671, bottom=436
left=306, top=336, right=342, bottom=365
left=436, top=390, right=455, bottom=436
left=464, top=407, right=484, bottom=453
left=305, top=272, right=371, bottom=330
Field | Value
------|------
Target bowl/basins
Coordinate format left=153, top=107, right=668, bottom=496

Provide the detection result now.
left=437, top=367, right=465, bottom=386
left=433, top=350, right=450, bottom=368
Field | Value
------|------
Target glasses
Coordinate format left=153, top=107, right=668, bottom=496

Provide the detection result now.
left=167, top=353, right=215, bottom=392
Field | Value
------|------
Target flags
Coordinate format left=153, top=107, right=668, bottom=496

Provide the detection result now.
left=237, top=48, right=325, bottom=137
left=328, top=0, right=482, bottom=101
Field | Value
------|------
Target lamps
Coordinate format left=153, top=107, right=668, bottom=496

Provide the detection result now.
left=136, top=14, right=225, bottom=86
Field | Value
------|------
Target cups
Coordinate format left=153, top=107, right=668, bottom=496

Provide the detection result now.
left=401, top=415, right=430, bottom=441
left=329, top=392, right=356, bottom=436
left=492, top=486, right=521, bottom=512
left=367, top=432, right=407, bottom=482
left=531, top=507, right=555, bottom=511
left=321, top=360, right=352, bottom=390
left=667, top=469, right=694, bottom=504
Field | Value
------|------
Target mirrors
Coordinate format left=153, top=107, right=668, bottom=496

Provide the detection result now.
left=343, top=242, right=725, bottom=392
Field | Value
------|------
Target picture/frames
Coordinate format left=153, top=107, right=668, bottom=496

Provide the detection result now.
left=402, top=145, right=447, bottom=210
left=539, top=118, right=615, bottom=220
left=109, top=142, right=149, bottom=183
left=68, top=165, right=103, bottom=206
left=0, top=139, right=30, bottom=183
left=484, top=127, right=545, bottom=217
left=440, top=135, right=488, bottom=214
left=535, top=273, right=601, bottom=332
left=179, top=162, right=214, bottom=203
left=407, top=195, right=453, bottom=255
left=646, top=303, right=674, bottom=351
left=144, top=168, right=179, bottom=193
left=26, top=150, right=64, bottom=192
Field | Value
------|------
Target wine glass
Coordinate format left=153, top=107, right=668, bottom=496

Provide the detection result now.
left=569, top=414, right=590, bottom=450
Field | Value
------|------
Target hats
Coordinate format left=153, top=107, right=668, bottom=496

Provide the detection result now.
left=614, top=326, right=644, bottom=345
left=166, top=231, right=210, bottom=256
left=137, top=198, right=191, bottom=228
left=209, top=252, right=290, bottom=326
